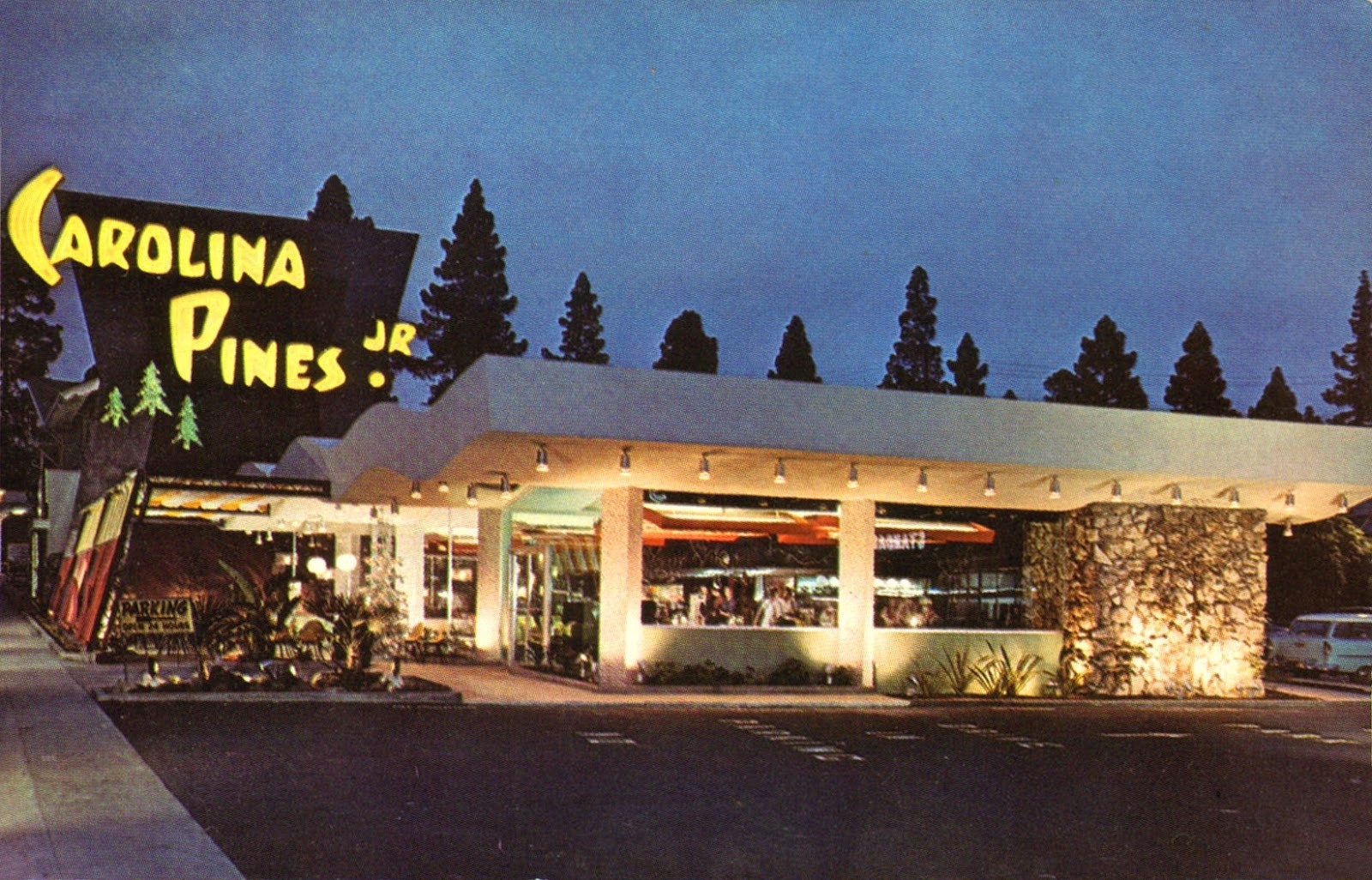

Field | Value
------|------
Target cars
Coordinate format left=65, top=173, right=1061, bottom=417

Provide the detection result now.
left=1276, top=612, right=1372, bottom=681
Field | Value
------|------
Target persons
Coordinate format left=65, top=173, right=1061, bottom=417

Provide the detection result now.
left=643, top=568, right=944, bottom=628
